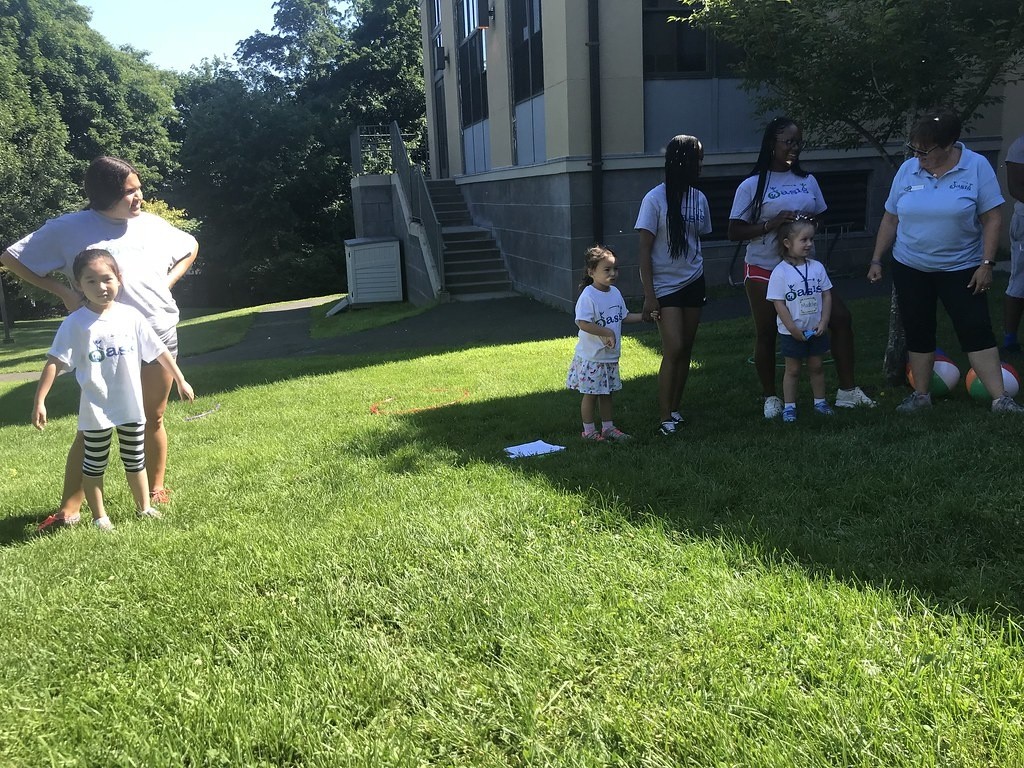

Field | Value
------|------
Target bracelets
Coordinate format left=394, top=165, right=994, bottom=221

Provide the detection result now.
left=982, top=259, right=996, bottom=267
left=870, top=260, right=884, bottom=268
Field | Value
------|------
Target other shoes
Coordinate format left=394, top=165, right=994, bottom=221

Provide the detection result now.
left=93, top=517, right=118, bottom=534
left=999, top=343, right=1020, bottom=355
left=136, top=508, right=162, bottom=520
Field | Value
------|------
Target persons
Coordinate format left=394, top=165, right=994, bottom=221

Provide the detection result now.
left=765, top=212, right=835, bottom=422
left=723, top=118, right=876, bottom=418
left=864, top=113, right=1024, bottom=414
left=0, top=157, right=198, bottom=534
left=1000, top=136, right=1024, bottom=356
left=30, top=248, right=194, bottom=529
left=632, top=137, right=713, bottom=437
left=562, top=246, right=661, bottom=441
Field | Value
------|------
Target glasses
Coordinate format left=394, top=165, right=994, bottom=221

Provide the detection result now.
left=906, top=141, right=939, bottom=156
left=776, top=139, right=806, bottom=150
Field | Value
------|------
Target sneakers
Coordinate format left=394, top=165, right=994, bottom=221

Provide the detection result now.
left=657, top=419, right=676, bottom=435
left=814, top=401, right=834, bottom=417
left=895, top=391, right=933, bottom=414
left=670, top=410, right=684, bottom=425
left=835, top=387, right=880, bottom=409
left=35, top=512, right=81, bottom=533
left=582, top=431, right=612, bottom=445
left=781, top=406, right=797, bottom=423
left=764, top=396, right=783, bottom=419
left=991, top=391, right=1024, bottom=414
left=150, top=488, right=174, bottom=507
left=602, top=426, right=631, bottom=440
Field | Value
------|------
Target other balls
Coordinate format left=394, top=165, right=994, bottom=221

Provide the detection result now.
left=966, top=356, right=1020, bottom=403
left=903, top=348, right=961, bottom=394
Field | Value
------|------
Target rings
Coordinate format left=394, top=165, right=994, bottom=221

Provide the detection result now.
left=985, top=288, right=987, bottom=289
left=988, top=287, right=990, bottom=289
left=981, top=289, right=985, bottom=291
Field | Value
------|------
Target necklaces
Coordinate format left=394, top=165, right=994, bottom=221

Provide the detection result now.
left=932, top=171, right=938, bottom=177
